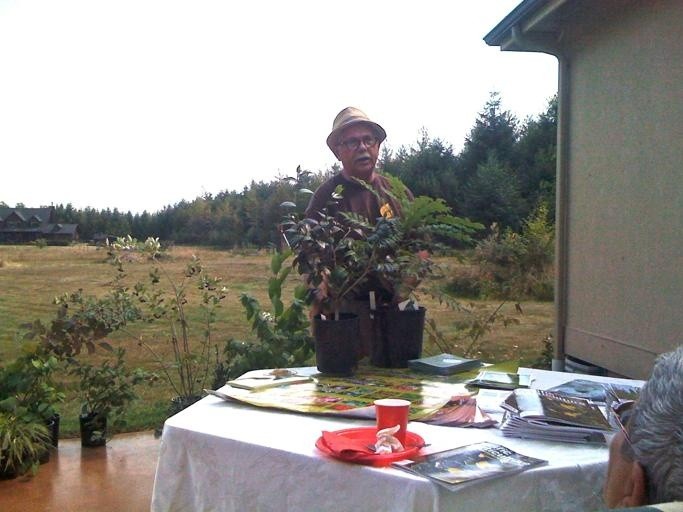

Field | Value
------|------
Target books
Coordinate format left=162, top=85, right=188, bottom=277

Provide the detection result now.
left=390, top=440, right=548, bottom=493
left=499, top=382, right=638, bottom=444
left=409, top=351, right=484, bottom=376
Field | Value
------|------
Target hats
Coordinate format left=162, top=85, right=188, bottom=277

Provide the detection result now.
left=326, top=107, right=387, bottom=161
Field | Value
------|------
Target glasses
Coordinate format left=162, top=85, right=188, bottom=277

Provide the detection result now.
left=334, top=135, right=379, bottom=152
left=610, top=399, right=643, bottom=462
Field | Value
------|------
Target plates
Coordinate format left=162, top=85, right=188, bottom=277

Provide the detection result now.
left=315, top=427, right=426, bottom=465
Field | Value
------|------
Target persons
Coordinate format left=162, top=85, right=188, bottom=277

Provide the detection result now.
left=601, top=346, right=683, bottom=508
left=303, top=107, right=428, bottom=315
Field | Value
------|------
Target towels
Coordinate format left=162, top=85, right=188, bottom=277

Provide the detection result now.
left=320, top=430, right=374, bottom=459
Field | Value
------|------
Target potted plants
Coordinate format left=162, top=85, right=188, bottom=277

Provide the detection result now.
left=0, top=352, right=52, bottom=465
left=26, top=345, right=61, bottom=451
left=339, top=167, right=486, bottom=370
left=61, top=351, right=159, bottom=449
left=0, top=417, right=52, bottom=482
left=273, top=164, right=373, bottom=378
left=103, top=227, right=228, bottom=416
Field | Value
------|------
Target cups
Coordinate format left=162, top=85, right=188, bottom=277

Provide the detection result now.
left=375, top=398, right=411, bottom=448
